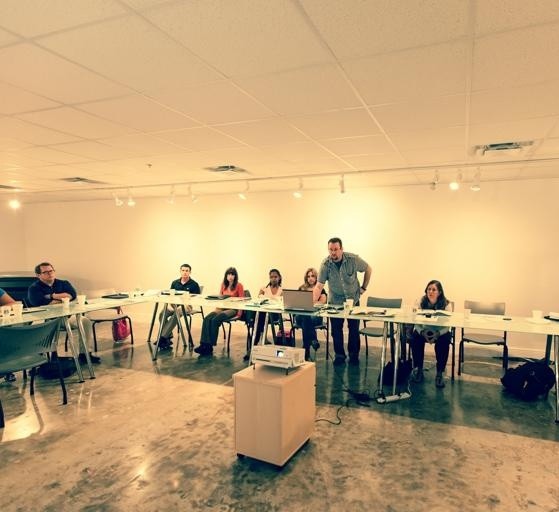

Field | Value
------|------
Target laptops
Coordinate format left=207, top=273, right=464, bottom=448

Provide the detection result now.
left=282, top=288, right=321, bottom=313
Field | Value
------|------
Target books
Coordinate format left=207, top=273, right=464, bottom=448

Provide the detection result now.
left=416, top=308, right=451, bottom=316
left=349, top=306, right=386, bottom=315
left=160, top=289, right=190, bottom=295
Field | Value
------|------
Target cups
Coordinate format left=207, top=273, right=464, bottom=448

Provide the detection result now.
left=532, top=310, right=544, bottom=319
left=1, top=306, right=12, bottom=318
left=343, top=299, right=354, bottom=313
left=359, top=294, right=367, bottom=309
left=77, top=295, right=86, bottom=306
left=128, top=285, right=283, bottom=308
left=11, top=303, right=23, bottom=319
left=463, top=308, right=472, bottom=320
left=61, top=297, right=70, bottom=307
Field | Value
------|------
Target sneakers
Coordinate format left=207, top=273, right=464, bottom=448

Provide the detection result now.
left=348, top=357, right=359, bottom=364
left=78, top=352, right=100, bottom=364
left=154, top=336, right=173, bottom=347
left=194, top=344, right=213, bottom=355
left=312, top=341, right=320, bottom=351
left=5, top=373, right=15, bottom=382
left=334, top=355, right=345, bottom=364
left=435, top=375, right=445, bottom=387
left=412, top=368, right=423, bottom=383
left=243, top=355, right=249, bottom=360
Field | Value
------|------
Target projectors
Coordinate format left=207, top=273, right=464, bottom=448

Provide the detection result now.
left=250, top=345, right=306, bottom=369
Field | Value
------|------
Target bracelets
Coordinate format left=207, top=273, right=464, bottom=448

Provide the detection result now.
left=361, top=287, right=366, bottom=291
left=49, top=292, right=54, bottom=299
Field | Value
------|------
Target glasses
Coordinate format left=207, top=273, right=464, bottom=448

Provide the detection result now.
left=42, top=270, right=54, bottom=274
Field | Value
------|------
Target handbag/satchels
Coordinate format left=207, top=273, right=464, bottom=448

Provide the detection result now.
left=112, top=306, right=131, bottom=341
left=378, top=359, right=412, bottom=384
left=500, top=359, right=556, bottom=399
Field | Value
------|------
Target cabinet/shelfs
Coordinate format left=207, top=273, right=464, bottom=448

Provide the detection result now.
left=232, top=363, right=317, bottom=471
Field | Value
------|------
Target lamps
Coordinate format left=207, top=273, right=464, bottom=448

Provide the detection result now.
left=111, top=188, right=138, bottom=208
left=291, top=177, right=306, bottom=199
left=164, top=185, right=201, bottom=207
left=337, top=173, right=348, bottom=197
left=236, top=181, right=252, bottom=201
left=427, top=168, right=483, bottom=194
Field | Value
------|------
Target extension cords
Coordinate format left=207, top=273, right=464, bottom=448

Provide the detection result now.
left=376, top=392, right=410, bottom=403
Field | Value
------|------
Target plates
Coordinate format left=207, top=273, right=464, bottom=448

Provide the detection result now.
left=525, top=317, right=552, bottom=324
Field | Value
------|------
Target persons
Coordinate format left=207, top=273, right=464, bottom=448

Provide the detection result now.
left=193, top=266, right=245, bottom=356
left=242, top=269, right=285, bottom=359
left=27, top=262, right=101, bottom=376
left=411, top=280, right=452, bottom=388
left=313, top=237, right=372, bottom=364
left=293, top=268, right=328, bottom=362
left=154, top=263, right=199, bottom=348
left=0, top=286, right=18, bottom=383
